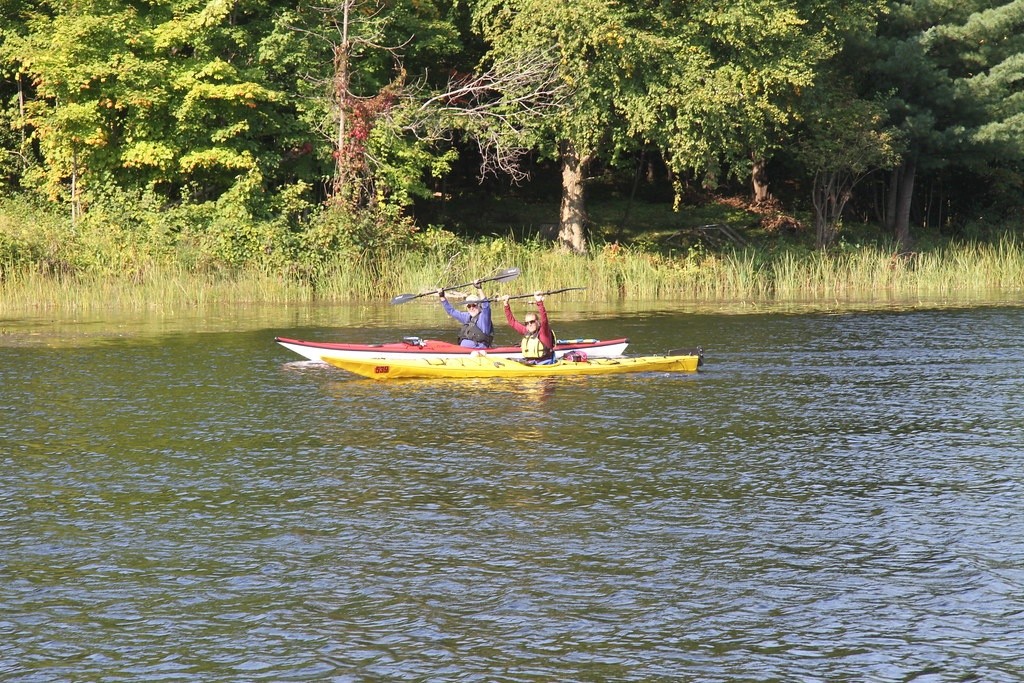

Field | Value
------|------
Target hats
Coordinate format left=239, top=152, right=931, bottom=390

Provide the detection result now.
left=466, top=295, right=480, bottom=305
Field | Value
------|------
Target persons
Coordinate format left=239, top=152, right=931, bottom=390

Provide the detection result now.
left=438, top=279, right=494, bottom=349
left=502, top=291, right=556, bottom=365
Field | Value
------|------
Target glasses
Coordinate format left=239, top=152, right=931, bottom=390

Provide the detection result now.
left=525, top=318, right=538, bottom=326
left=466, top=303, right=479, bottom=308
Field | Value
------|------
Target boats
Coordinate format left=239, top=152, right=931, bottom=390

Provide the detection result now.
left=321, top=352, right=705, bottom=382
left=273, top=336, right=631, bottom=367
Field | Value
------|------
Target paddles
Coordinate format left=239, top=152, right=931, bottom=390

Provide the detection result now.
left=389, top=268, right=520, bottom=307
left=458, top=285, right=590, bottom=306
